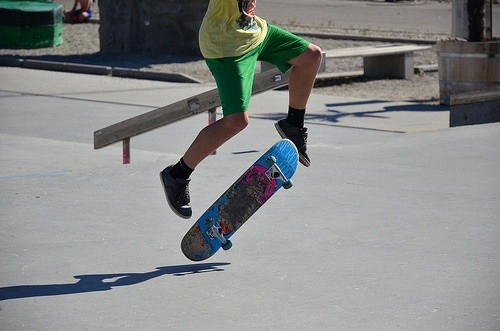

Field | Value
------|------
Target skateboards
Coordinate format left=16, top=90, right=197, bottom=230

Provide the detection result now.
left=180, top=139, right=299, bottom=262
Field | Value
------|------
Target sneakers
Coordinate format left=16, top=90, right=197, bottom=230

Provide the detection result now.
left=274, top=118, right=312, bottom=167
left=159, top=164, right=192, bottom=219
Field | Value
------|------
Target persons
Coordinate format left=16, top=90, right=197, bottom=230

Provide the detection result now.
left=63, top=0, right=93, bottom=22
left=158, top=0, right=323, bottom=220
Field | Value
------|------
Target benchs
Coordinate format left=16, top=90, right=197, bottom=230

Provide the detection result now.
left=259, top=43, right=433, bottom=88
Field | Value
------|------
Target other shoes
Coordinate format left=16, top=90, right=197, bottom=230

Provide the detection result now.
left=63, top=16, right=69, bottom=22
